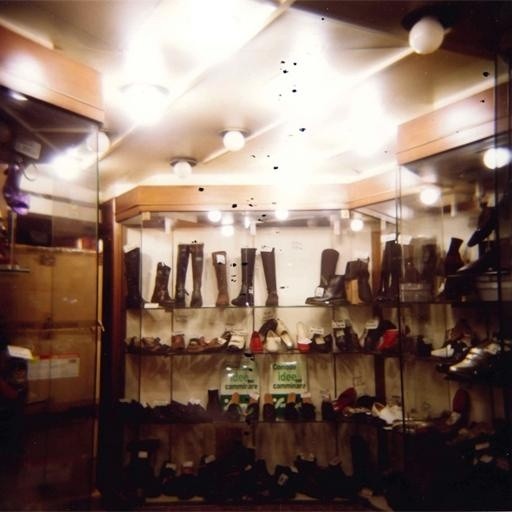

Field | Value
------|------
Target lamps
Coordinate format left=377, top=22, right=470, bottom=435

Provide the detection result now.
left=218, top=129, right=251, bottom=152
left=402, top=7, right=448, bottom=56
left=170, top=159, right=197, bottom=177
left=84, top=126, right=110, bottom=155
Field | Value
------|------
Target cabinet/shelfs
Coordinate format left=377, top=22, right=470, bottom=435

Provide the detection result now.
left=104, top=81, right=511, bottom=512
left=1, top=23, right=104, bottom=511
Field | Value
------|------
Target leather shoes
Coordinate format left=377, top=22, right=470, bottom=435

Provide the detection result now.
left=457, top=250, right=496, bottom=275
left=467, top=207, right=496, bottom=247
